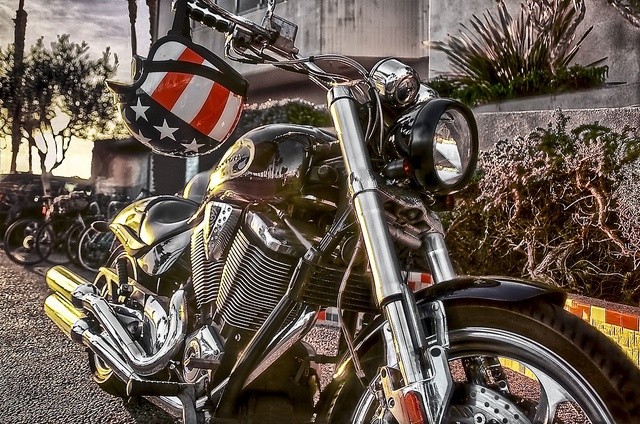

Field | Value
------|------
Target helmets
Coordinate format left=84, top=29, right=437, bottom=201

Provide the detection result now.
left=104, top=34, right=249, bottom=158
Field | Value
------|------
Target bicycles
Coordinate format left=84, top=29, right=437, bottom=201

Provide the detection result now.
left=1, top=175, right=152, bottom=272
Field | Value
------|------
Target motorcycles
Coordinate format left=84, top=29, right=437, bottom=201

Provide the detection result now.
left=43, top=0, right=639, bottom=423
